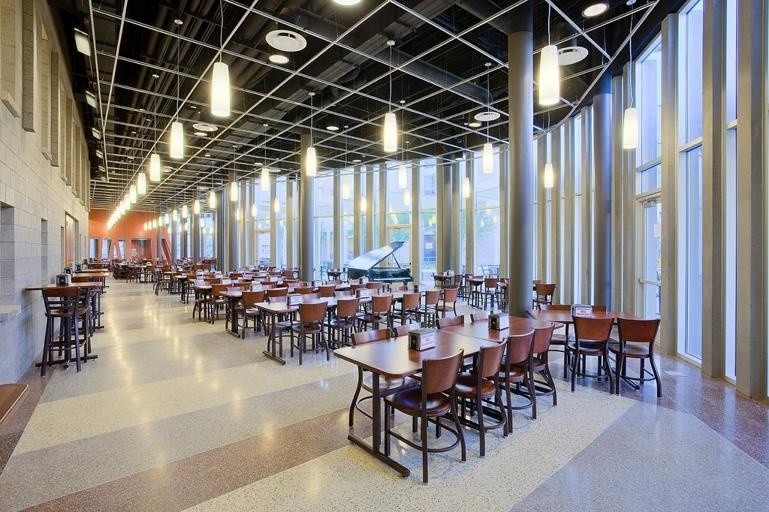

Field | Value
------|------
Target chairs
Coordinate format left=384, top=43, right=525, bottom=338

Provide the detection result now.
left=112, top=258, right=335, bottom=364
left=25, top=259, right=110, bottom=377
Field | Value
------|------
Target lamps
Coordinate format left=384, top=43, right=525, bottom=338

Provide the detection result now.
left=70, top=1, right=238, bottom=232
